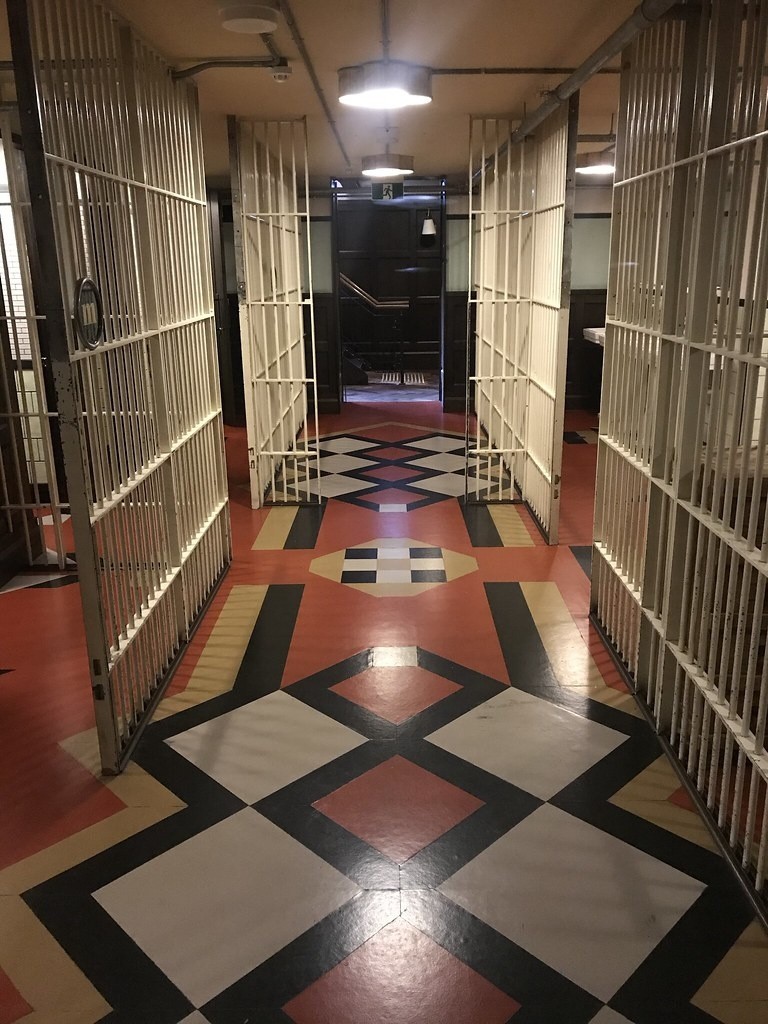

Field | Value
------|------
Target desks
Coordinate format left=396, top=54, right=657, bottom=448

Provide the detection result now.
left=583, top=328, right=725, bottom=370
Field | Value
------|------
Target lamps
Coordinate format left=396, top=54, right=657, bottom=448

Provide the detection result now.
left=336, top=60, right=434, bottom=110
left=575, top=151, right=615, bottom=175
left=422, top=209, right=436, bottom=236
left=361, top=154, right=414, bottom=176
